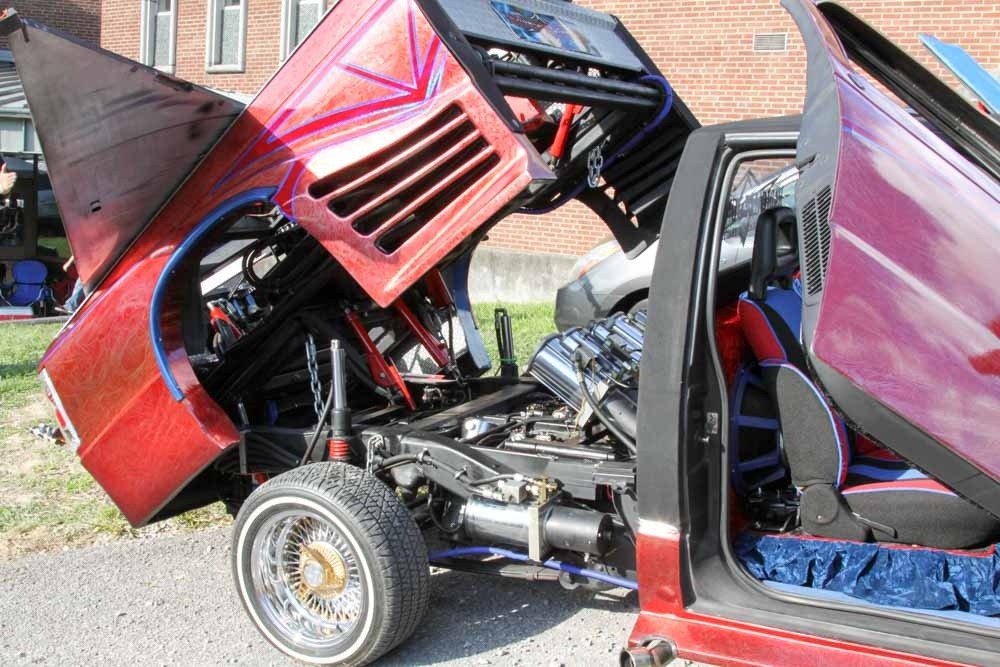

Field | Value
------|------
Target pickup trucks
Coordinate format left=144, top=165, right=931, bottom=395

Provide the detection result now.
left=0, top=0, right=1000, bottom=665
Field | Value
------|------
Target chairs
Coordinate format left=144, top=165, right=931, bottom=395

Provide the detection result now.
left=736, top=204, right=992, bottom=549
left=0, top=175, right=85, bottom=317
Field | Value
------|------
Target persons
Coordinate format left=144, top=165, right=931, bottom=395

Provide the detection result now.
left=54, top=255, right=83, bottom=314
left=0, top=162, right=16, bottom=210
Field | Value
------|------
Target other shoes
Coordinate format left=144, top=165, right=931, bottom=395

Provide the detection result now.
left=54, top=305, right=74, bottom=313
